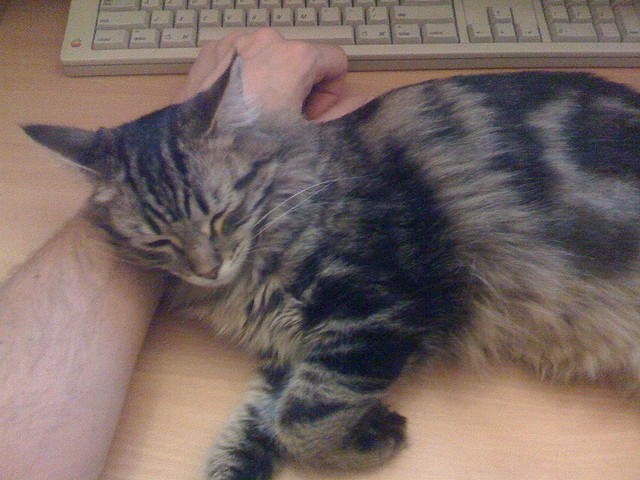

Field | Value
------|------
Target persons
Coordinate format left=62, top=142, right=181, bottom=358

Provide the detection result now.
left=1, top=25, right=376, bottom=479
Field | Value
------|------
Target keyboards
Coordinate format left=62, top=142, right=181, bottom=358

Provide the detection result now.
left=60, top=0, right=639, bottom=77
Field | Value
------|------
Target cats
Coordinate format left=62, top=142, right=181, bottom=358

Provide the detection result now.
left=16, top=44, right=640, bottom=480
